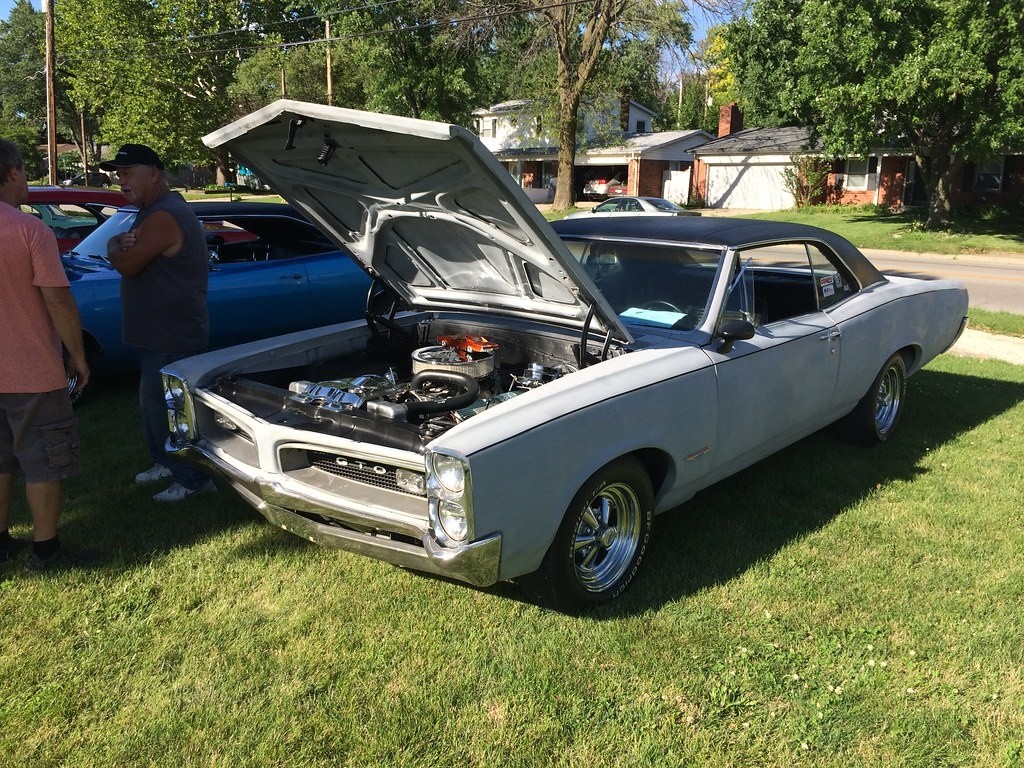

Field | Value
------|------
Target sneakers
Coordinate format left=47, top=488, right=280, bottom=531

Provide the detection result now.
left=135, top=463, right=174, bottom=484
left=151, top=478, right=218, bottom=505
left=0, top=538, right=30, bottom=563
left=22, top=542, right=111, bottom=576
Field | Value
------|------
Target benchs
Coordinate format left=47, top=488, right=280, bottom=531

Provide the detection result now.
left=241, top=239, right=342, bottom=262
left=636, top=265, right=836, bottom=328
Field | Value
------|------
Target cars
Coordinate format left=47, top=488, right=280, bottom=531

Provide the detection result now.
left=158, top=100, right=968, bottom=610
left=18, top=186, right=131, bottom=254
left=59, top=201, right=391, bottom=407
left=62, top=172, right=113, bottom=189
left=584, top=178, right=629, bottom=196
left=564, top=196, right=701, bottom=221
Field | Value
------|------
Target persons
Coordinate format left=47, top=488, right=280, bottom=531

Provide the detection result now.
left=0, top=138, right=92, bottom=575
left=107, top=144, right=216, bottom=504
left=550, top=174, right=557, bottom=191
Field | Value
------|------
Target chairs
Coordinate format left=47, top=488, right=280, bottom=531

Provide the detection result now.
left=239, top=240, right=272, bottom=262
left=205, top=233, right=224, bottom=264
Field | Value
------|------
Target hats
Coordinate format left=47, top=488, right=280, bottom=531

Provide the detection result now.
left=99, top=143, right=166, bottom=171
